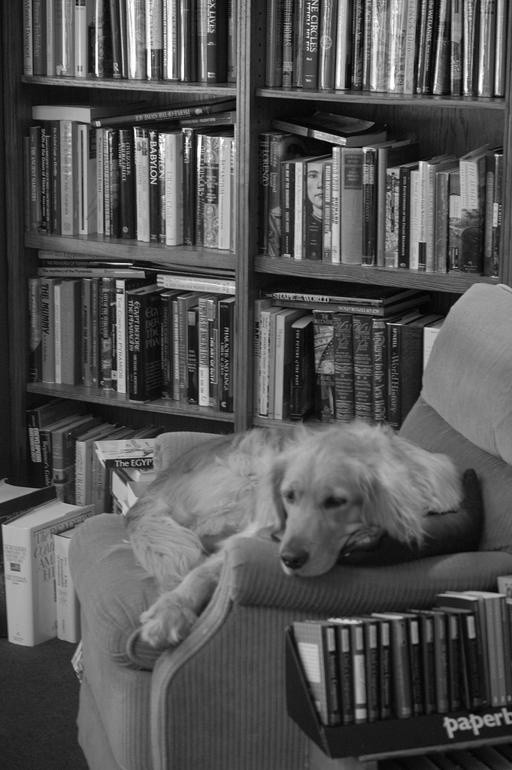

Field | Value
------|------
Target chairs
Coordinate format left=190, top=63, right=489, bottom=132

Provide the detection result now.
left=69, top=275, right=510, bottom=769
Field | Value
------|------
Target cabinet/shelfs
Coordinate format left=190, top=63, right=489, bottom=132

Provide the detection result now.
left=0, top=1, right=511, bottom=483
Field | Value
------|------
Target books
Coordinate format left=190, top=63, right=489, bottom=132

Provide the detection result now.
left=291, top=571, right=512, bottom=728
left=1, top=431, right=157, bottom=649
left=13, top=0, right=512, bottom=431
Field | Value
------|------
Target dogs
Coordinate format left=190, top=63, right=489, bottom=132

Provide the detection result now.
left=124, top=418, right=464, bottom=648
